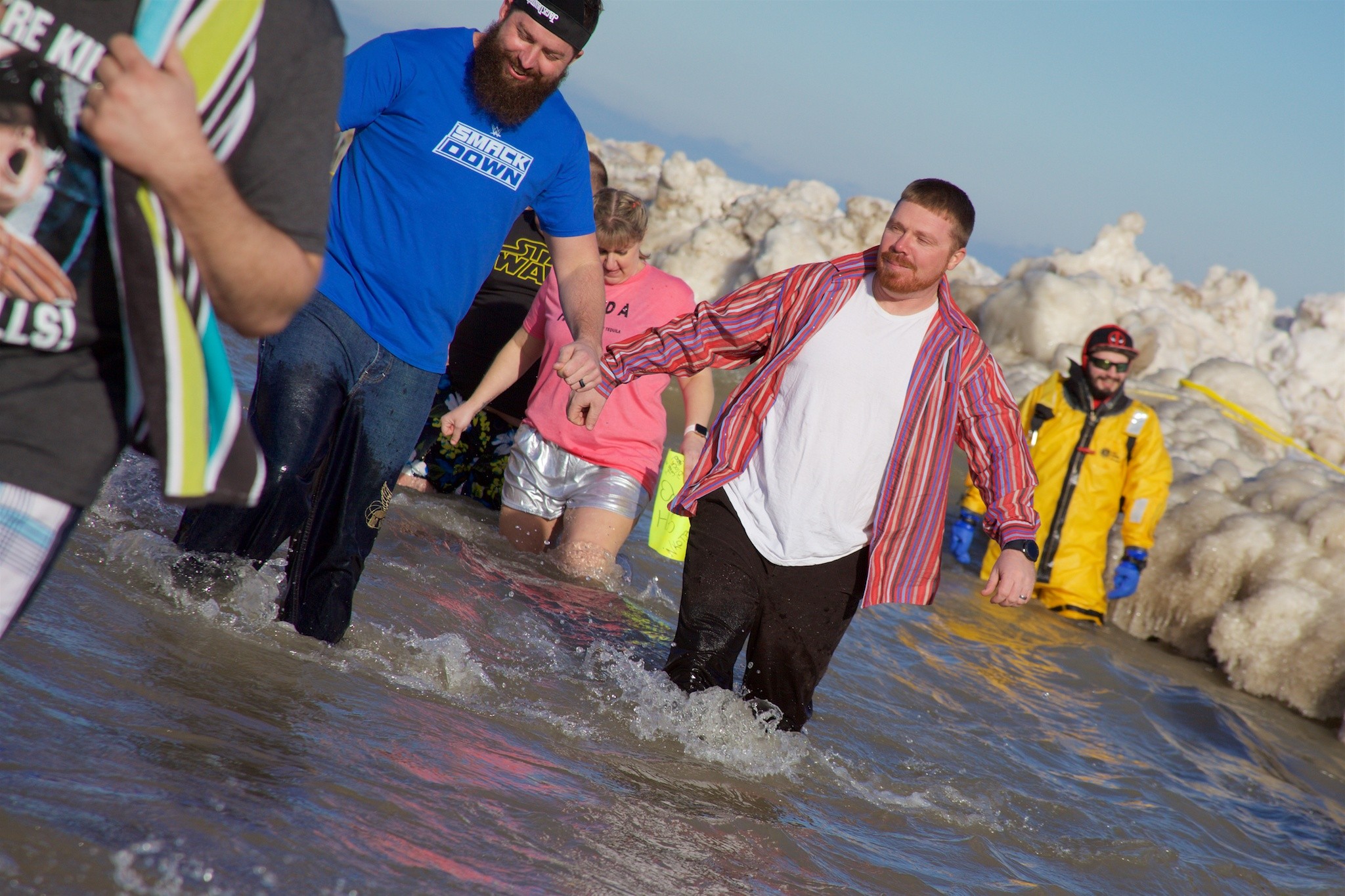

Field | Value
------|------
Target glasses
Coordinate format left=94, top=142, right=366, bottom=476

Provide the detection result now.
left=1087, top=355, right=1129, bottom=372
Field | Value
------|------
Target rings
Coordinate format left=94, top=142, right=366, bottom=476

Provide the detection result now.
left=579, top=380, right=585, bottom=387
left=1020, top=595, right=1027, bottom=600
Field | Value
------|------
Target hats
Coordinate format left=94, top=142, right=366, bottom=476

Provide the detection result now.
left=1081, top=324, right=1138, bottom=367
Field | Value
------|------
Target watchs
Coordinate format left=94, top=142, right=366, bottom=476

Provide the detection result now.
left=1003, top=540, right=1039, bottom=562
left=684, top=423, right=708, bottom=439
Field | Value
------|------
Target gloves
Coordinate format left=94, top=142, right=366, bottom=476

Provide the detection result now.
left=951, top=507, right=984, bottom=564
left=1107, top=546, right=1148, bottom=599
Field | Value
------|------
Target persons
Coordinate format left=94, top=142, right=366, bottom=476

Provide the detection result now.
left=948, top=324, right=1174, bottom=627
left=172, top=0, right=607, bottom=644
left=565, top=178, right=1042, bottom=733
left=0, top=0, right=346, bottom=642
left=398, top=150, right=608, bottom=510
left=440, top=189, right=714, bottom=575
